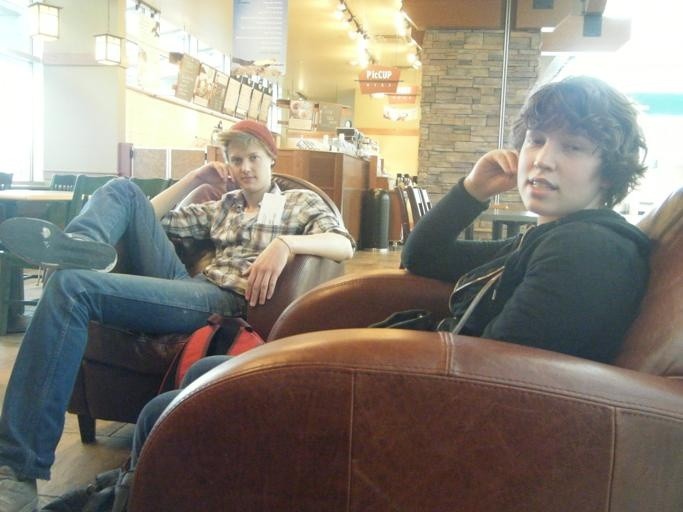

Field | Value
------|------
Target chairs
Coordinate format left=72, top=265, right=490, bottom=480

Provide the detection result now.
left=64, top=173, right=349, bottom=445
left=129, top=175, right=173, bottom=199
left=42, top=174, right=76, bottom=221
left=0, top=176, right=113, bottom=335
left=124, top=188, right=682, bottom=511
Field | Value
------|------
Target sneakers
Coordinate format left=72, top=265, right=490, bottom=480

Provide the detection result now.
left=0, top=466, right=37, bottom=512
left=0, top=217, right=118, bottom=274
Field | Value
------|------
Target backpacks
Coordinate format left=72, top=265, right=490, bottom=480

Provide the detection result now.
left=154, top=313, right=266, bottom=394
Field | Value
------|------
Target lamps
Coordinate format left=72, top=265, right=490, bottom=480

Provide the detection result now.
left=92, top=1, right=124, bottom=67
left=25, top=2, right=64, bottom=41
left=393, top=11, right=421, bottom=74
left=333, top=1, right=371, bottom=51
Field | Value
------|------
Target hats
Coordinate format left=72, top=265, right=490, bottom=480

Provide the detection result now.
left=223, top=121, right=277, bottom=167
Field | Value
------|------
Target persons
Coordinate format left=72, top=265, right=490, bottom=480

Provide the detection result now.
left=0, top=119, right=358, bottom=512
left=39, top=73, right=653, bottom=511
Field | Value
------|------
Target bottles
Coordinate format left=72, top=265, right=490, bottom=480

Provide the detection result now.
left=297, top=135, right=307, bottom=150
left=395, top=173, right=411, bottom=187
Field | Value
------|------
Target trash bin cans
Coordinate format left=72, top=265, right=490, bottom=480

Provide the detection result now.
left=361, top=187, right=391, bottom=252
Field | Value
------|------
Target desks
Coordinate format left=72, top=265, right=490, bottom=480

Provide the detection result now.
left=1, top=187, right=90, bottom=333
